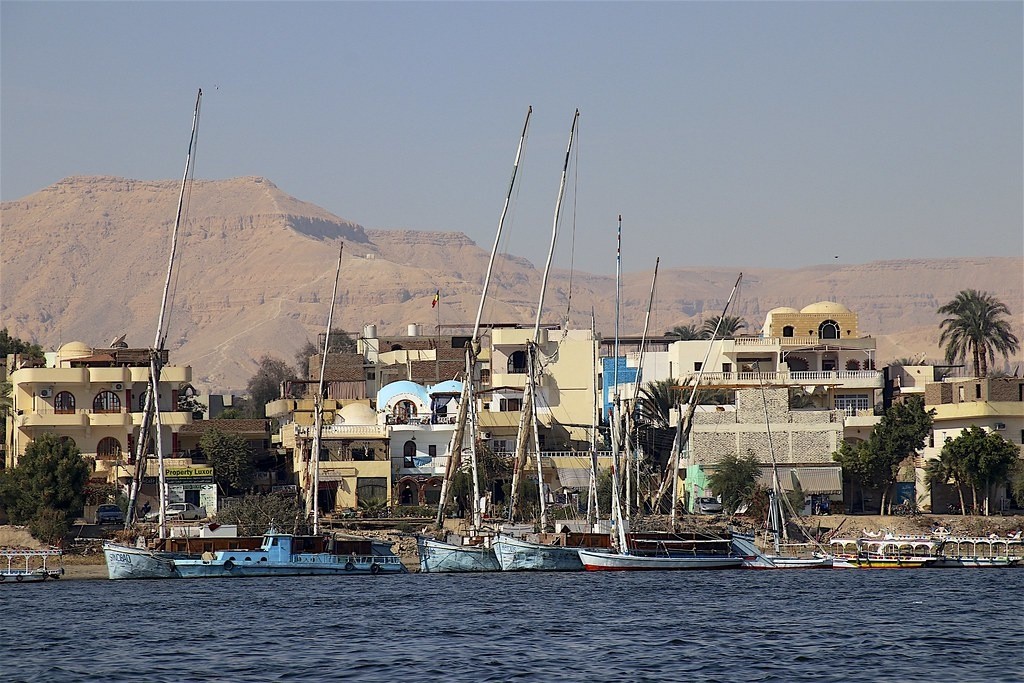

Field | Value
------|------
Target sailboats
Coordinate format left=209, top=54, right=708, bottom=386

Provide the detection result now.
left=100, top=85, right=1022, bottom=572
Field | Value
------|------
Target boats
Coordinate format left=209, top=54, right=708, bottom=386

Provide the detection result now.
left=0, top=545, right=65, bottom=583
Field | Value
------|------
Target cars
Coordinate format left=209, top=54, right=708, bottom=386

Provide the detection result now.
left=94, top=504, right=124, bottom=525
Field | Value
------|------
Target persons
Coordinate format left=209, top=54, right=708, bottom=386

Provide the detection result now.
left=454, top=487, right=468, bottom=519
left=141, top=500, right=151, bottom=523
left=644, top=497, right=653, bottom=515
left=399, top=484, right=415, bottom=506
left=675, top=499, right=684, bottom=516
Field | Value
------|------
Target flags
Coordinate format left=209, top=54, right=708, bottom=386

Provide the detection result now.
left=432, top=290, right=440, bottom=309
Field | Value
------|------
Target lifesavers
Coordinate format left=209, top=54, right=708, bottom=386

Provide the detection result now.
left=224, top=560, right=234, bottom=571
left=370, top=563, right=379, bottom=574
left=345, top=562, right=354, bottom=572
